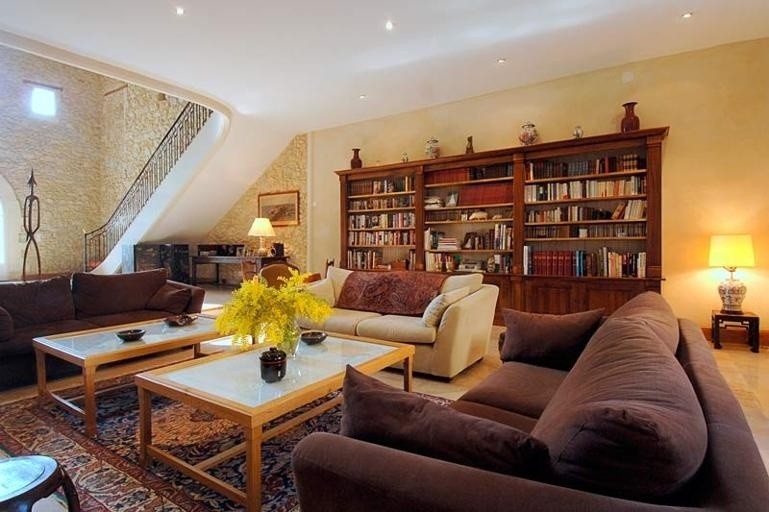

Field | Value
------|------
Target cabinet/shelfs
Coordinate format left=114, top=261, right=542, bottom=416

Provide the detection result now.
left=420, top=145, right=521, bottom=272
left=522, top=125, right=671, bottom=276
left=334, top=160, right=421, bottom=270
left=133, top=242, right=190, bottom=283
left=481, top=274, right=522, bottom=325
left=522, top=278, right=664, bottom=317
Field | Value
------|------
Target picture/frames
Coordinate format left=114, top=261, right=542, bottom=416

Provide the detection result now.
left=257, top=188, right=301, bottom=227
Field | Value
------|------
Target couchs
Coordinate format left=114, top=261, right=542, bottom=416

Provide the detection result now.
left=295, top=288, right=767, bottom=512
left=1, top=269, right=206, bottom=397
left=281, top=265, right=501, bottom=383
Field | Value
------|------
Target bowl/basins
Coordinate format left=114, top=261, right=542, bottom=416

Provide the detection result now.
left=300, top=332, right=328, bottom=345
left=117, top=330, right=146, bottom=341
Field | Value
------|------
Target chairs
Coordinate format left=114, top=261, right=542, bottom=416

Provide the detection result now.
left=256, top=263, right=302, bottom=292
left=239, top=258, right=259, bottom=291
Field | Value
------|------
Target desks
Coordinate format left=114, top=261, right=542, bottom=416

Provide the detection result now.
left=189, top=254, right=289, bottom=282
left=711, top=312, right=757, bottom=350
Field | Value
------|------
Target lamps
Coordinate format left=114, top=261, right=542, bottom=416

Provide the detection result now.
left=246, top=217, right=276, bottom=255
left=706, top=235, right=757, bottom=312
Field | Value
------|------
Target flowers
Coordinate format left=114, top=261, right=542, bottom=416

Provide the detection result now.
left=215, top=274, right=332, bottom=352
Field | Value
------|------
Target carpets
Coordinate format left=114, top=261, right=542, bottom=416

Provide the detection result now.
left=1, top=372, right=457, bottom=512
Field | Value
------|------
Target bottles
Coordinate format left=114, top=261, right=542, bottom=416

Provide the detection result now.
left=259, top=347, right=288, bottom=385
left=425, top=135, right=441, bottom=159
left=519, top=120, right=537, bottom=146
left=573, top=126, right=584, bottom=138
left=399, top=151, right=408, bottom=163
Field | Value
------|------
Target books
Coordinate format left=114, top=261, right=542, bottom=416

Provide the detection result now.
left=347, top=174, right=416, bottom=272
left=425, top=251, right=446, bottom=273
left=423, top=162, right=513, bottom=184
left=523, top=153, right=647, bottom=279
left=437, top=222, right=513, bottom=250
left=456, top=181, right=513, bottom=206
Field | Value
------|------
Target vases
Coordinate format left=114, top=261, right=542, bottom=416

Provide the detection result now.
left=273, top=325, right=301, bottom=358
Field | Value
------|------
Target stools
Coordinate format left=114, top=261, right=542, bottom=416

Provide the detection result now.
left=1, top=455, right=83, bottom=512
